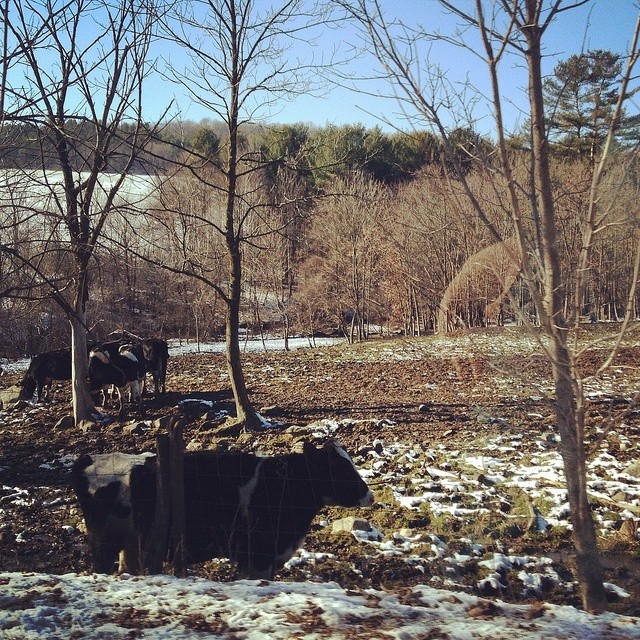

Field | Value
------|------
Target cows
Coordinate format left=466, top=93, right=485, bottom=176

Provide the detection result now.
left=70, top=438, right=375, bottom=581
left=87, top=339, right=146, bottom=413
left=20, top=343, right=72, bottom=404
left=132, top=339, right=169, bottom=394
